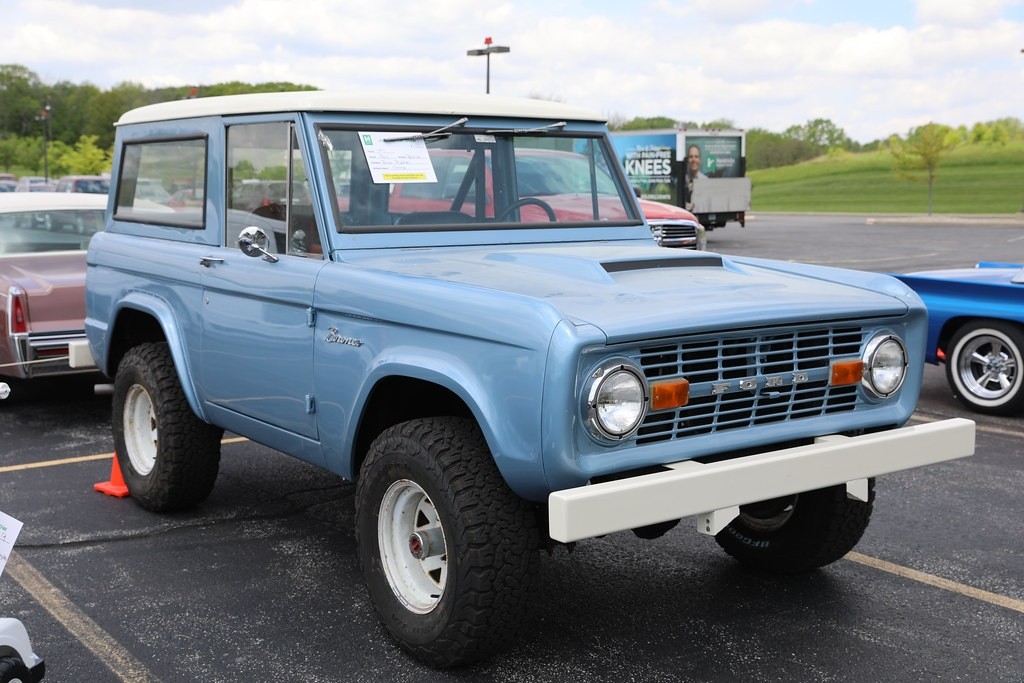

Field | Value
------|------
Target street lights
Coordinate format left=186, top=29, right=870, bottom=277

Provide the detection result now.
left=467, top=46, right=510, bottom=95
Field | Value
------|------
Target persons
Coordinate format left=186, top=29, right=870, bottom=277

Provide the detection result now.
left=682, top=144, right=710, bottom=203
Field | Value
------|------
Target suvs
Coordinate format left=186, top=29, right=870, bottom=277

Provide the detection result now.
left=79, top=85, right=977, bottom=671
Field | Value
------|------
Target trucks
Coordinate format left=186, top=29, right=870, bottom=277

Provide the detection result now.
left=568, top=120, right=750, bottom=232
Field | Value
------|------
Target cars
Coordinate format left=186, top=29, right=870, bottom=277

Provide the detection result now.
left=884, top=260, right=1024, bottom=417
left=0, top=188, right=186, bottom=380
left=2, top=170, right=352, bottom=236
left=325, top=148, right=706, bottom=255
